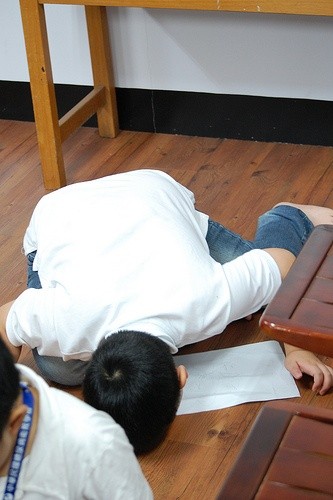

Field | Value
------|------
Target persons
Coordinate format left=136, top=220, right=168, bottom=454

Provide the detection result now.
left=0, top=334, right=153, bottom=500
left=0, top=168, right=333, bottom=455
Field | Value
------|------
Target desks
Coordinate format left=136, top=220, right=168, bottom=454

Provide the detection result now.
left=18, top=0, right=333, bottom=192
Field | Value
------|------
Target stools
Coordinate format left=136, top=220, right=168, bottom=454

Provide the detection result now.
left=212, top=400, right=333, bottom=500
left=259, top=224, right=333, bottom=359
